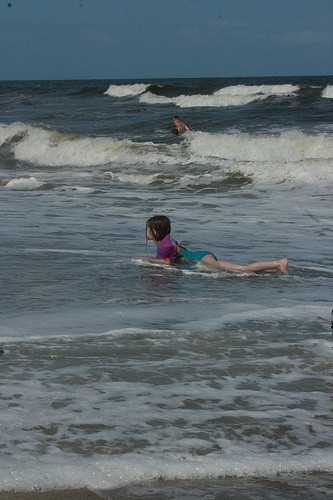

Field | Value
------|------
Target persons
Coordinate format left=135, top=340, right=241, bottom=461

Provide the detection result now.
left=141, top=215, right=287, bottom=277
left=171, top=115, right=189, bottom=136
